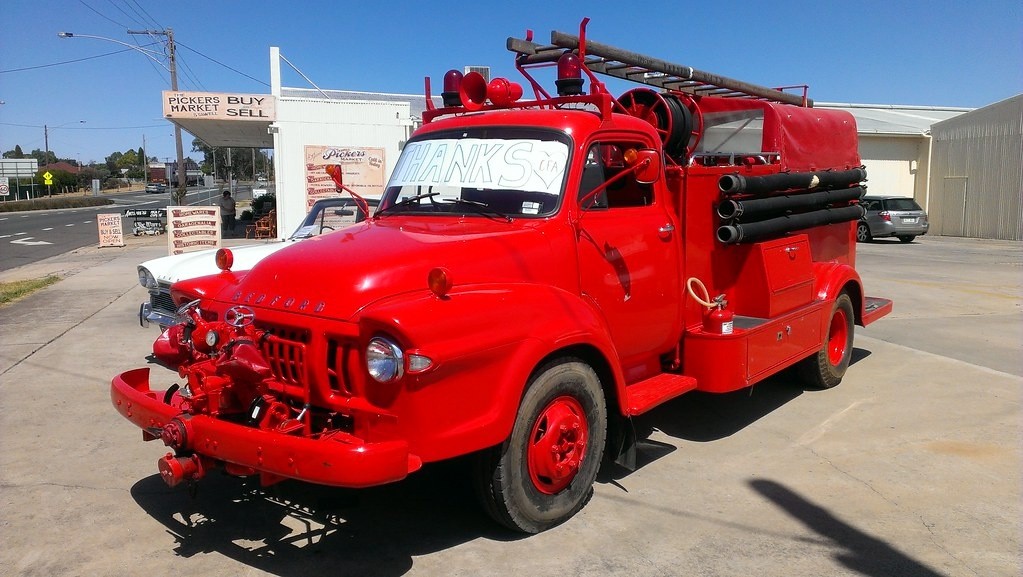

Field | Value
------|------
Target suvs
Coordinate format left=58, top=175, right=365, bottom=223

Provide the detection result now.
left=856, top=194, right=931, bottom=244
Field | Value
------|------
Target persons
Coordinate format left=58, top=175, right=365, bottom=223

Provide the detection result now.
left=219, top=190, right=237, bottom=235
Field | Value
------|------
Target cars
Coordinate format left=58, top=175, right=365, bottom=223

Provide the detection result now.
left=144, top=182, right=166, bottom=194
left=132, top=193, right=418, bottom=337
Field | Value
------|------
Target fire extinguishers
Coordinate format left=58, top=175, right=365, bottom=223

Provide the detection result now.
left=701, top=293, right=734, bottom=335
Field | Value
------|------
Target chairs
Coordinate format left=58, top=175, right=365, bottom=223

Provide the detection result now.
left=246, top=210, right=277, bottom=239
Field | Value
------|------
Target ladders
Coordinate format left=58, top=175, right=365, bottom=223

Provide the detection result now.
left=503, top=30, right=817, bottom=111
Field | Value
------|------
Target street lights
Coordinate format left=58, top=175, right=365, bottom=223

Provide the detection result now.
left=44, top=120, right=87, bottom=198
left=57, top=32, right=187, bottom=199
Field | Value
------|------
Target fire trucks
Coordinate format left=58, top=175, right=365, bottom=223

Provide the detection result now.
left=106, top=12, right=893, bottom=537
